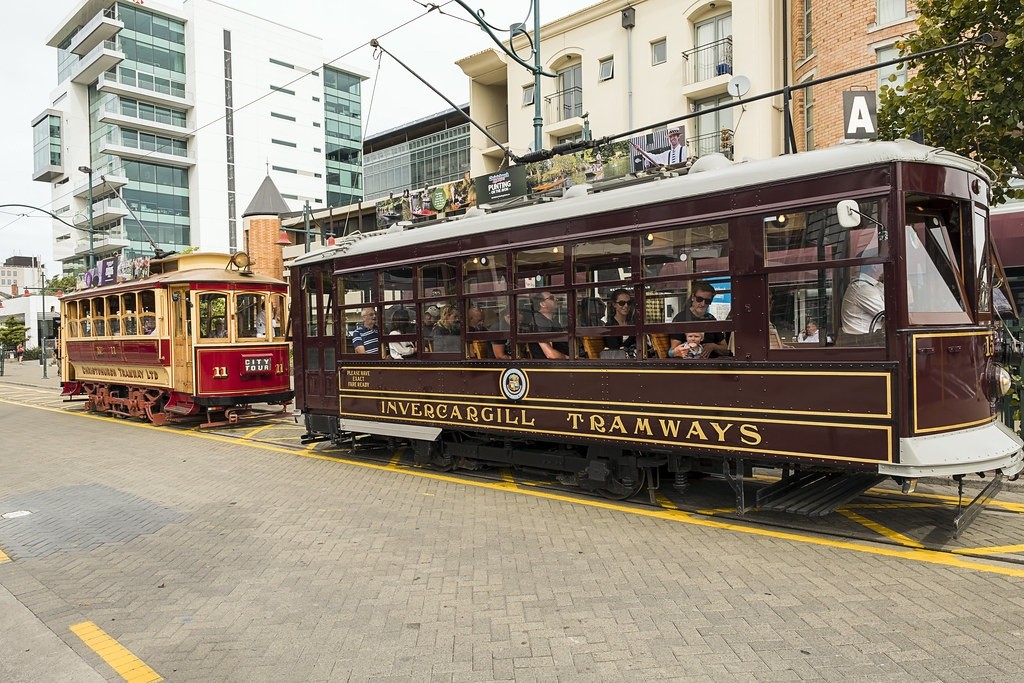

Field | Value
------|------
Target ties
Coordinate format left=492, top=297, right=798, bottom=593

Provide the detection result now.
left=672, top=151, right=675, bottom=163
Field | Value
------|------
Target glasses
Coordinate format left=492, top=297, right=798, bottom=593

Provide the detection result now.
left=545, top=295, right=554, bottom=301
left=694, top=295, right=712, bottom=304
left=616, top=300, right=631, bottom=306
left=363, top=313, right=376, bottom=316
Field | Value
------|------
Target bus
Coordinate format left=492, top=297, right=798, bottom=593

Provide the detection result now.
left=284, top=39, right=1024, bottom=541
left=57, top=174, right=294, bottom=429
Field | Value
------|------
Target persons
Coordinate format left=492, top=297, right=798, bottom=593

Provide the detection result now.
left=352, top=308, right=378, bottom=353
left=209, top=319, right=228, bottom=338
left=683, top=332, right=704, bottom=359
left=431, top=305, right=461, bottom=351
left=580, top=297, right=606, bottom=327
left=17, top=344, right=24, bottom=364
left=670, top=283, right=730, bottom=359
left=256, top=301, right=280, bottom=337
left=798, top=321, right=831, bottom=342
left=389, top=309, right=417, bottom=360
left=841, top=248, right=885, bottom=334
left=424, top=306, right=440, bottom=349
left=528, top=291, right=569, bottom=359
left=469, top=305, right=488, bottom=332
left=636, top=128, right=687, bottom=165
left=54, top=342, right=61, bottom=377
left=487, top=305, right=523, bottom=360
left=603, top=289, right=636, bottom=356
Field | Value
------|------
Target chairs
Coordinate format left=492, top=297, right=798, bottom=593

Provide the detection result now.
left=429, top=321, right=782, bottom=361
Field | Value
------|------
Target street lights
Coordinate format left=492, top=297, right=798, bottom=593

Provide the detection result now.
left=78, top=165, right=95, bottom=269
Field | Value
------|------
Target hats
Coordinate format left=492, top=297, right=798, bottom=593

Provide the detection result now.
left=666, top=129, right=682, bottom=138
left=425, top=306, right=440, bottom=317
